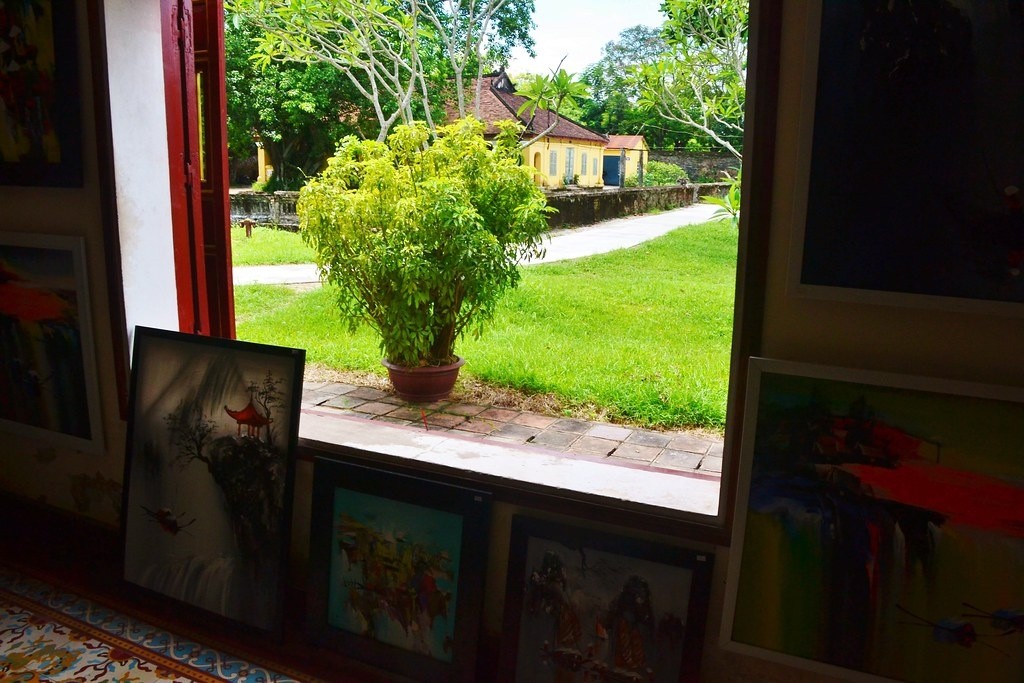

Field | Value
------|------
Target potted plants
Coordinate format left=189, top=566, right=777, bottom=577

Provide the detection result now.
left=563, top=173, right=580, bottom=190
left=294, top=90, right=560, bottom=404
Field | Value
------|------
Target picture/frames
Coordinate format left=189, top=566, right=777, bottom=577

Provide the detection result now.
left=497, top=514, right=716, bottom=683
left=718, top=354, right=1023, bottom=683
left=112, top=325, right=305, bottom=650
left=1, top=0, right=82, bottom=188
left=1, top=232, right=107, bottom=456
left=785, top=0, right=1023, bottom=320
left=303, top=453, right=495, bottom=683
